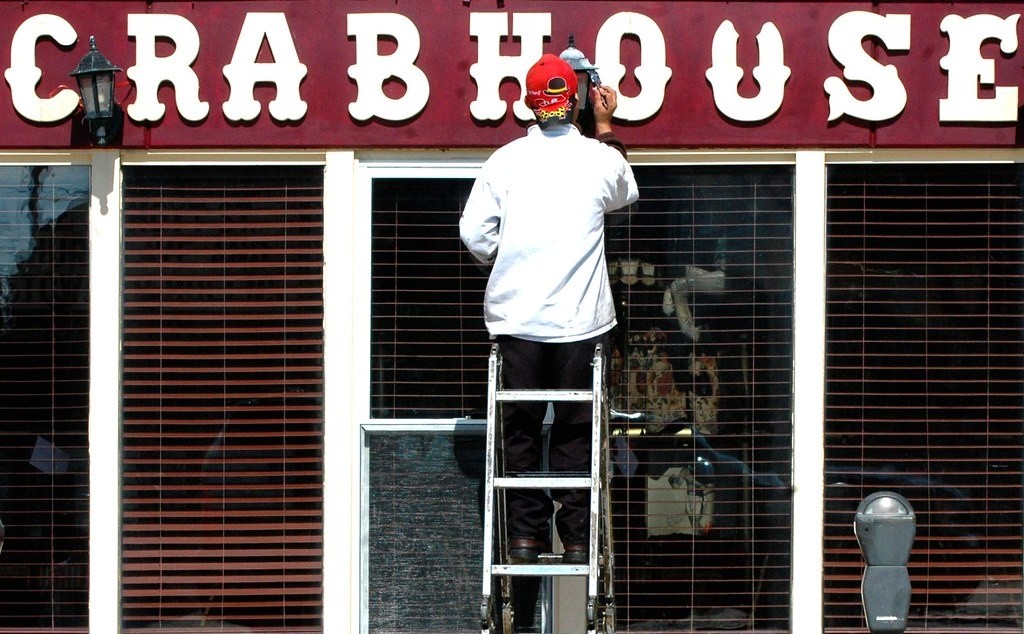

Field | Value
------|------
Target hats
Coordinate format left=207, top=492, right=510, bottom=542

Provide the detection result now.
left=526, top=54, right=579, bottom=113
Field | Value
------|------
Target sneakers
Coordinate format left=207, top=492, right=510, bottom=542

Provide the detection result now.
left=563, top=543, right=589, bottom=563
left=509, top=537, right=541, bottom=560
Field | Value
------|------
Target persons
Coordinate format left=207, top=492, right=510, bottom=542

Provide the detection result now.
left=458, top=52, right=638, bottom=563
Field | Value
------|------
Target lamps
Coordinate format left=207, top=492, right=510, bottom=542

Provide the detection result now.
left=67, top=35, right=124, bottom=147
left=559, top=31, right=600, bottom=133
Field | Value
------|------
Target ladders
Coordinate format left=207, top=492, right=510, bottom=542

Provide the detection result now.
left=477, top=341, right=620, bottom=633
